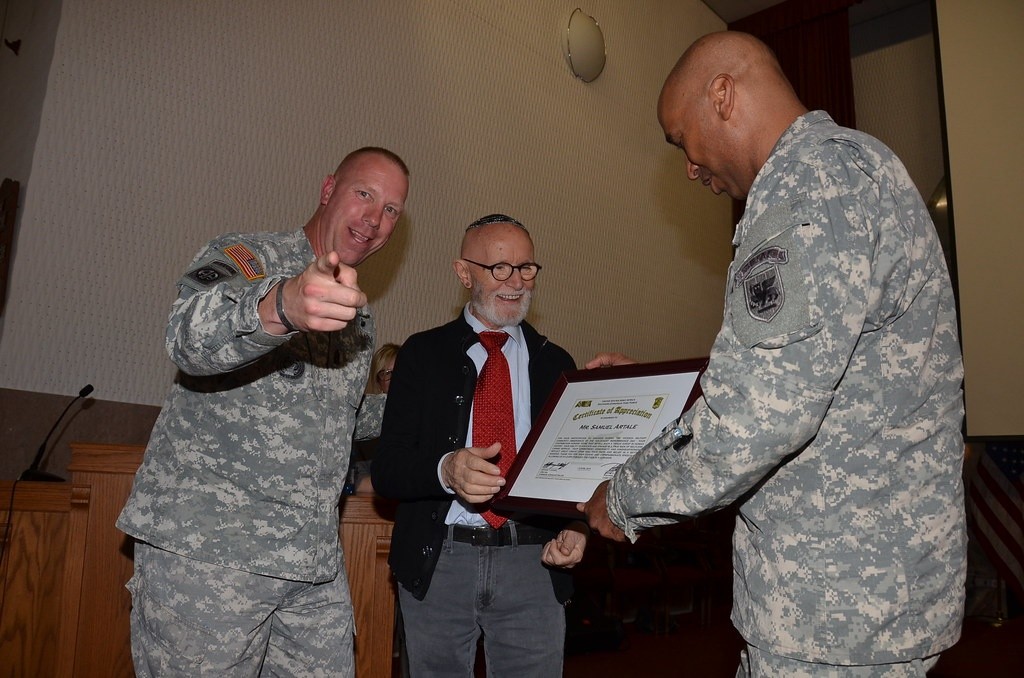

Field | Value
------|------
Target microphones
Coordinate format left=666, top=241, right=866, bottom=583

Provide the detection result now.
left=18, top=384, right=95, bottom=481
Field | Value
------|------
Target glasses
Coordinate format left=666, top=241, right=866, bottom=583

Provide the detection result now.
left=461, top=256, right=543, bottom=281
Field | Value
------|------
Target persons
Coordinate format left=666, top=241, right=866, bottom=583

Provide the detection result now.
left=575, top=28, right=965, bottom=678
left=112, top=147, right=413, bottom=678
left=368, top=212, right=576, bottom=678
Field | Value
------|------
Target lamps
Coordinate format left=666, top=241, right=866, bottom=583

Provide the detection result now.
left=561, top=8, right=607, bottom=83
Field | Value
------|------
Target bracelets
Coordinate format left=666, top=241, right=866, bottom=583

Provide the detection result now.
left=272, top=276, right=294, bottom=331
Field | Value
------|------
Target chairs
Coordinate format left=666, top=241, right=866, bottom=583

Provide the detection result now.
left=580, top=511, right=735, bottom=637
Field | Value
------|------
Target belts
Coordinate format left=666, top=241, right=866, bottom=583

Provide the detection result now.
left=443, top=523, right=548, bottom=546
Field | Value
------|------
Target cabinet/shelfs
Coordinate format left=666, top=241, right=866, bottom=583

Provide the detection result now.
left=55, top=441, right=395, bottom=678
left=0, top=480, right=72, bottom=678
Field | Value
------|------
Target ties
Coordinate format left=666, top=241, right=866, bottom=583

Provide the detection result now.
left=472, top=330, right=517, bottom=530
